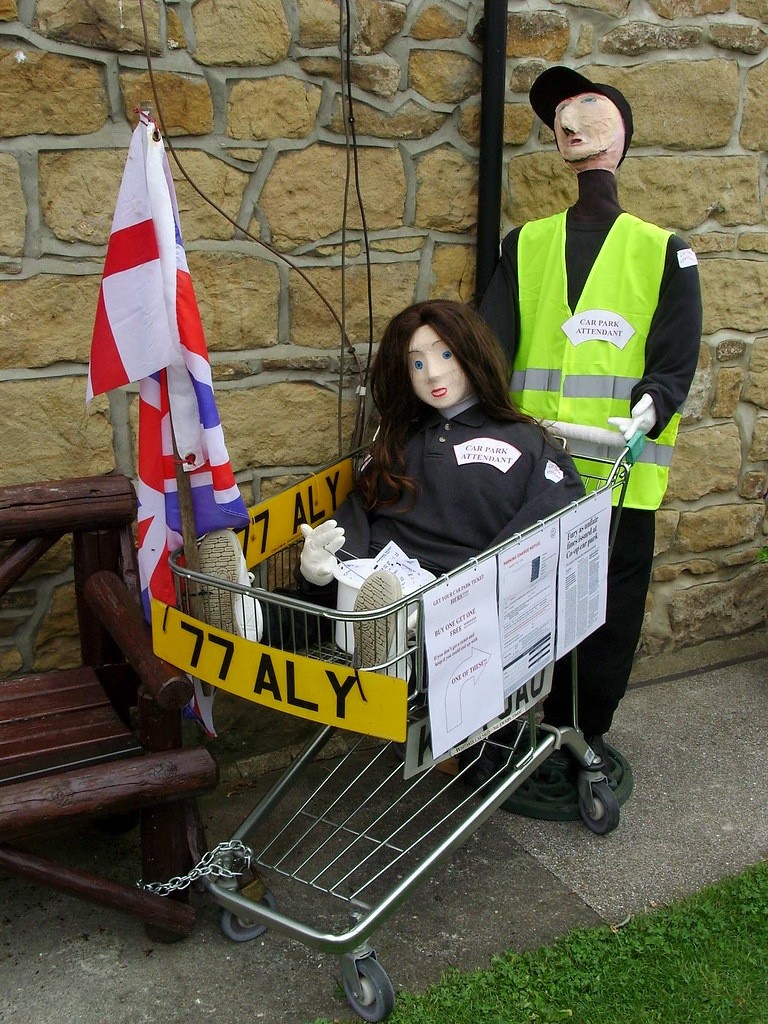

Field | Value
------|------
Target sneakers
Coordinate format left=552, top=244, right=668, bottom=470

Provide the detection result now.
left=542, top=743, right=570, bottom=770
left=349, top=569, right=413, bottom=682
left=583, top=733, right=610, bottom=776
left=197, top=528, right=264, bottom=642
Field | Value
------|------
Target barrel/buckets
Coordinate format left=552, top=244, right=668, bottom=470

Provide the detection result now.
left=332, top=558, right=438, bottom=656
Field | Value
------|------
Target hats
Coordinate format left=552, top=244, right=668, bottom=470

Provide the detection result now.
left=527, top=67, right=634, bottom=169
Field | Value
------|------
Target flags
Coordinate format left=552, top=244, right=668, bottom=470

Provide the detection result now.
left=86, top=105, right=253, bottom=738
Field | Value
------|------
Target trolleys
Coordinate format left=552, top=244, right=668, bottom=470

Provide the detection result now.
left=166, top=398, right=644, bottom=1024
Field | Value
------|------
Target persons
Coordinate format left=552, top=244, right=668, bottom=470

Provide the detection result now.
left=195, top=295, right=590, bottom=710
left=468, top=64, right=704, bottom=806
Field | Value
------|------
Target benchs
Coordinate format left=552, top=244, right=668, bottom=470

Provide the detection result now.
left=1, top=470, right=222, bottom=945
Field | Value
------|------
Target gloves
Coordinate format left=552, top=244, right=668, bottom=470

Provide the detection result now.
left=299, top=518, right=346, bottom=586
left=607, top=392, right=656, bottom=440
